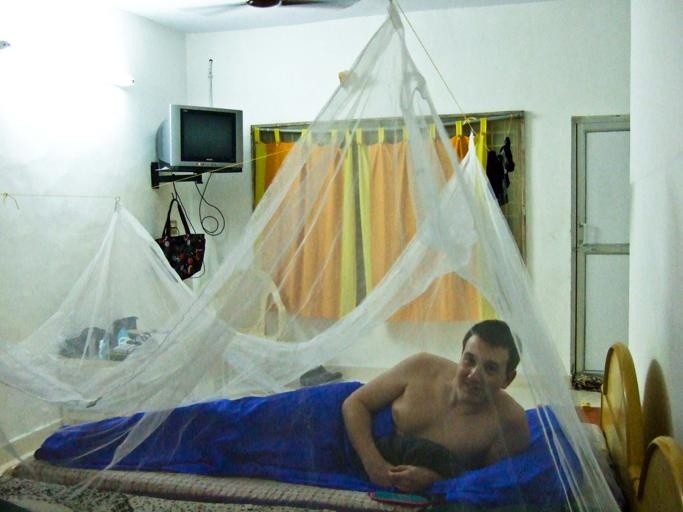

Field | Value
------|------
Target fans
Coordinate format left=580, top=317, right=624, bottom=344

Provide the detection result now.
left=183, top=1, right=357, bottom=12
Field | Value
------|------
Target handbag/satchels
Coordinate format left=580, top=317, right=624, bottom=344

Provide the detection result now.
left=155, top=199, right=205, bottom=279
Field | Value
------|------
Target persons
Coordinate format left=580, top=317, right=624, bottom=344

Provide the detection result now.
left=342, top=319, right=529, bottom=491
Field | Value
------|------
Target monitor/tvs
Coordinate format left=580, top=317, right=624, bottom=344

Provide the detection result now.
left=156, top=103, right=244, bottom=174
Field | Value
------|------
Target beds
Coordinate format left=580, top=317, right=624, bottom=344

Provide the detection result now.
left=1, top=342, right=683, bottom=512
